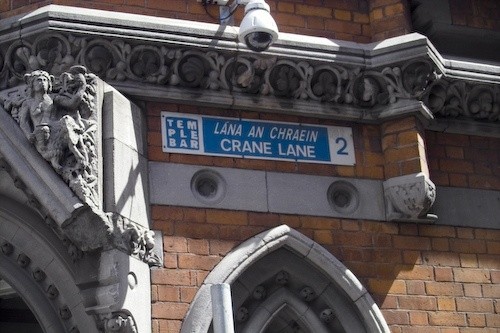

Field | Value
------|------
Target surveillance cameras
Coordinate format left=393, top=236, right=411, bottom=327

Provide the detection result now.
left=238, top=0, right=279, bottom=53
